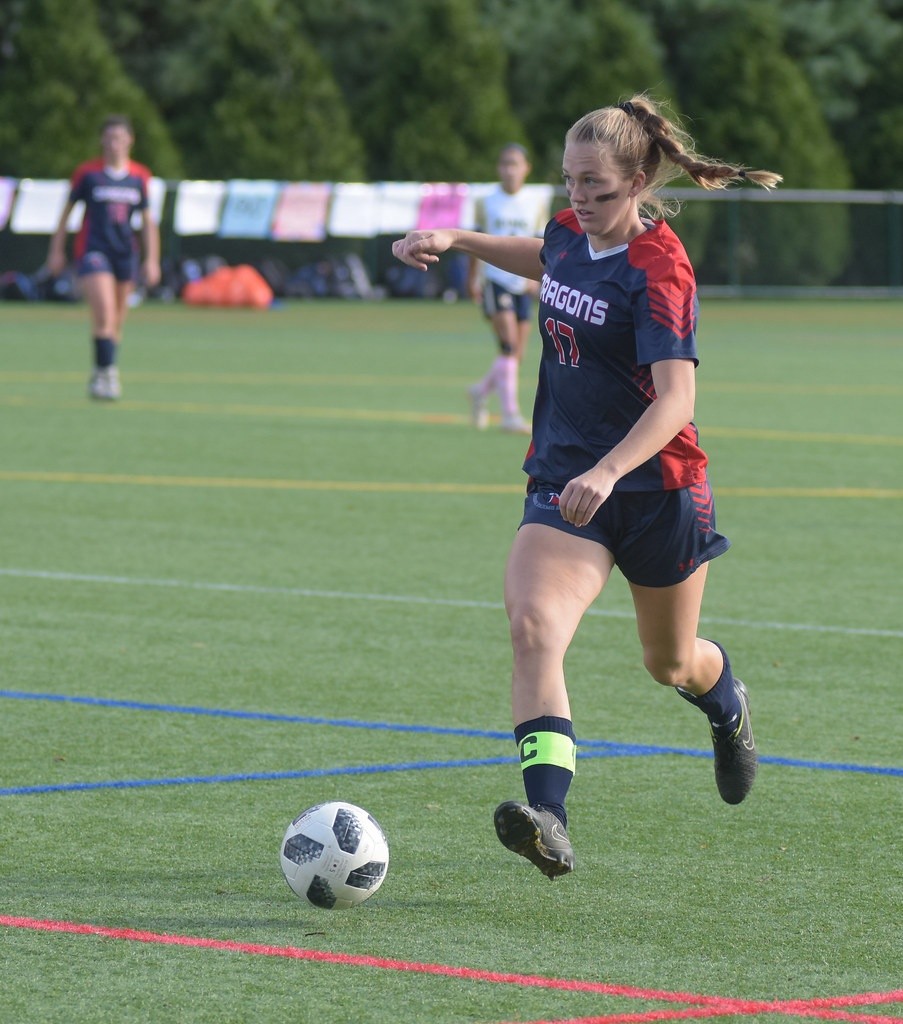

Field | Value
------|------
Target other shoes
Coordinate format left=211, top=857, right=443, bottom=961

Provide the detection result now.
left=468, top=385, right=488, bottom=431
left=503, top=418, right=532, bottom=436
left=89, top=363, right=122, bottom=400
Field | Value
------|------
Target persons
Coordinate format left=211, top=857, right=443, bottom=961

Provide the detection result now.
left=392, top=93, right=784, bottom=881
left=468, top=142, right=549, bottom=433
left=47, top=117, right=161, bottom=399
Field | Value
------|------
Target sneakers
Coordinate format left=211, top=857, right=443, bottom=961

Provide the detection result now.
left=493, top=802, right=574, bottom=880
left=707, top=677, right=758, bottom=805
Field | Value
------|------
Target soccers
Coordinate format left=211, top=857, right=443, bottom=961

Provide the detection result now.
left=279, top=800, right=390, bottom=911
